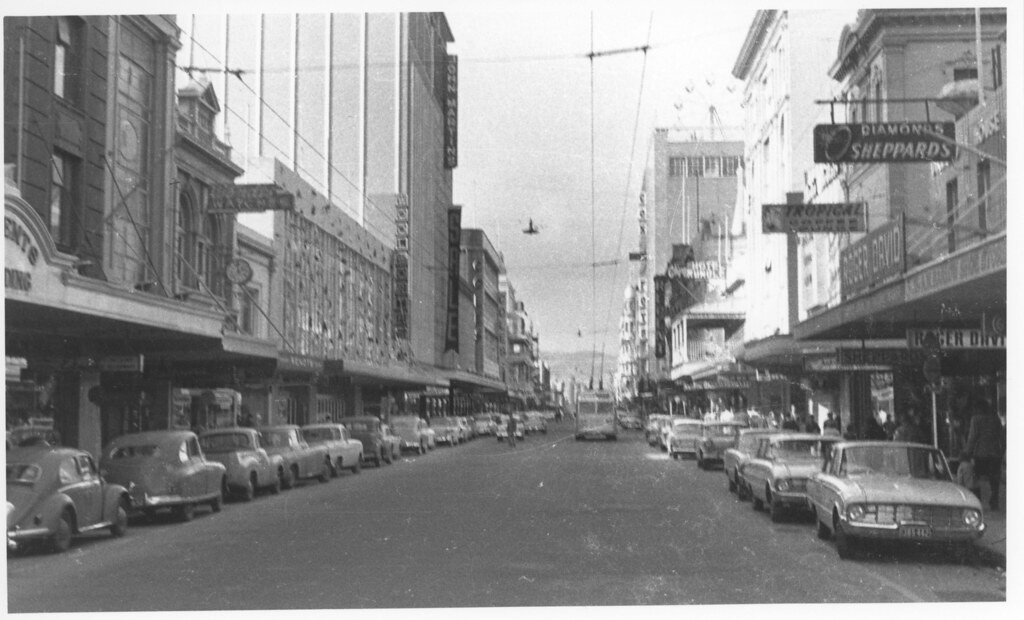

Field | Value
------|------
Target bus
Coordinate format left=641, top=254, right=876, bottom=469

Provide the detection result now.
left=575, top=389, right=617, bottom=438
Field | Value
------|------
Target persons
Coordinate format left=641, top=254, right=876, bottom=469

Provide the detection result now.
left=506, top=412, right=517, bottom=448
left=823, top=412, right=839, bottom=432
left=804, top=416, right=821, bottom=456
left=765, top=410, right=970, bottom=483
left=555, top=408, right=563, bottom=424
left=781, top=414, right=798, bottom=431
left=962, top=399, right=1001, bottom=510
left=889, top=414, right=927, bottom=443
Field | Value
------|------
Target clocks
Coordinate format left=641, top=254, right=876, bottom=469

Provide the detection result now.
left=118, top=118, right=139, bottom=160
left=225, top=258, right=252, bottom=284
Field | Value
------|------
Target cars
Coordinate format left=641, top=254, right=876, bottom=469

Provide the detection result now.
left=724, top=429, right=780, bottom=493
left=646, top=413, right=677, bottom=451
left=620, top=416, right=642, bottom=428
left=337, top=415, right=384, bottom=466
left=806, top=439, right=986, bottom=563
left=695, top=421, right=746, bottom=470
left=300, top=423, right=362, bottom=477
left=389, top=414, right=436, bottom=455
left=262, top=424, right=333, bottom=489
left=739, top=433, right=847, bottom=523
left=380, top=424, right=403, bottom=463
left=199, top=427, right=284, bottom=501
left=99, top=429, right=225, bottom=520
left=434, top=410, right=554, bottom=446
left=6, top=440, right=131, bottom=555
left=666, top=419, right=703, bottom=459
